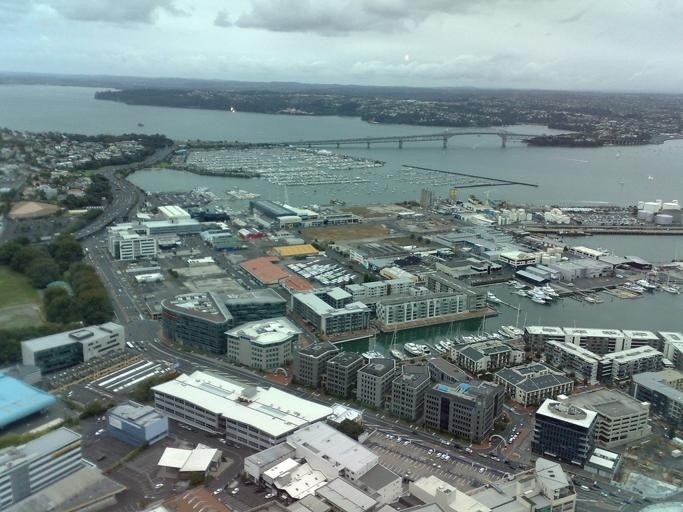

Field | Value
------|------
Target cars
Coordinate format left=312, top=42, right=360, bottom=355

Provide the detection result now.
left=383, top=422, right=530, bottom=483
left=255, top=488, right=266, bottom=493
left=47, top=340, right=151, bottom=387
left=154, top=484, right=163, bottom=490
left=213, top=487, right=222, bottom=495
left=95, top=429, right=106, bottom=436
left=568, top=472, right=655, bottom=506
left=559, top=256, right=568, bottom=261
left=567, top=282, right=574, bottom=287
left=264, top=493, right=276, bottom=500
left=176, top=422, right=240, bottom=448
left=231, top=488, right=239, bottom=495
left=244, top=480, right=253, bottom=486
left=96, top=455, right=106, bottom=462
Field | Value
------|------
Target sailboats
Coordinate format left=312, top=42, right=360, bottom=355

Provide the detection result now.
left=362, top=302, right=529, bottom=362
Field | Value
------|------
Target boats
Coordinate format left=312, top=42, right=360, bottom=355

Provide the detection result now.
left=581, top=271, right=682, bottom=304
left=484, top=277, right=558, bottom=307
